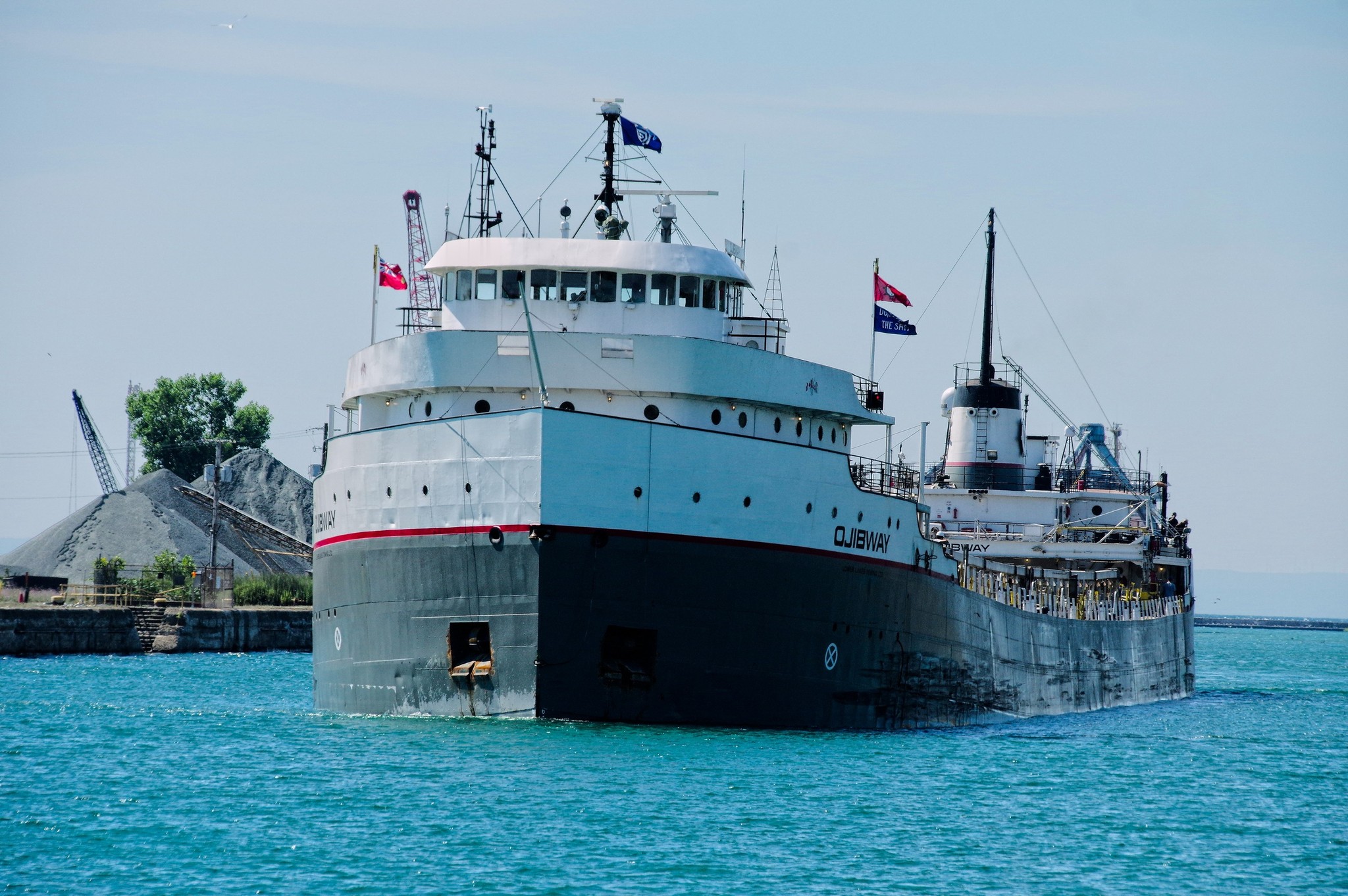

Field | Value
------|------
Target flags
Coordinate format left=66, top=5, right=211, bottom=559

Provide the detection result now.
left=620, top=115, right=662, bottom=153
left=874, top=273, right=913, bottom=307
left=379, top=256, right=407, bottom=290
left=874, top=303, right=917, bottom=335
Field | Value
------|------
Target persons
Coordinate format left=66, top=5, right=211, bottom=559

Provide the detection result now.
left=1168, top=512, right=1178, bottom=536
left=1163, top=575, right=1176, bottom=601
left=1116, top=567, right=1127, bottom=586
left=1174, top=519, right=1188, bottom=536
left=936, top=531, right=945, bottom=539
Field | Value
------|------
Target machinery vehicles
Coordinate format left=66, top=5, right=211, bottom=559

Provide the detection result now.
left=125, top=378, right=143, bottom=487
left=72, top=389, right=122, bottom=495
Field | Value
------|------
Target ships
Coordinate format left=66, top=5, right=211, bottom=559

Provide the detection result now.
left=311, top=99, right=1198, bottom=732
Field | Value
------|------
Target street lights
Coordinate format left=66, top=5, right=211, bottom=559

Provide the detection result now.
left=204, top=463, right=234, bottom=569
